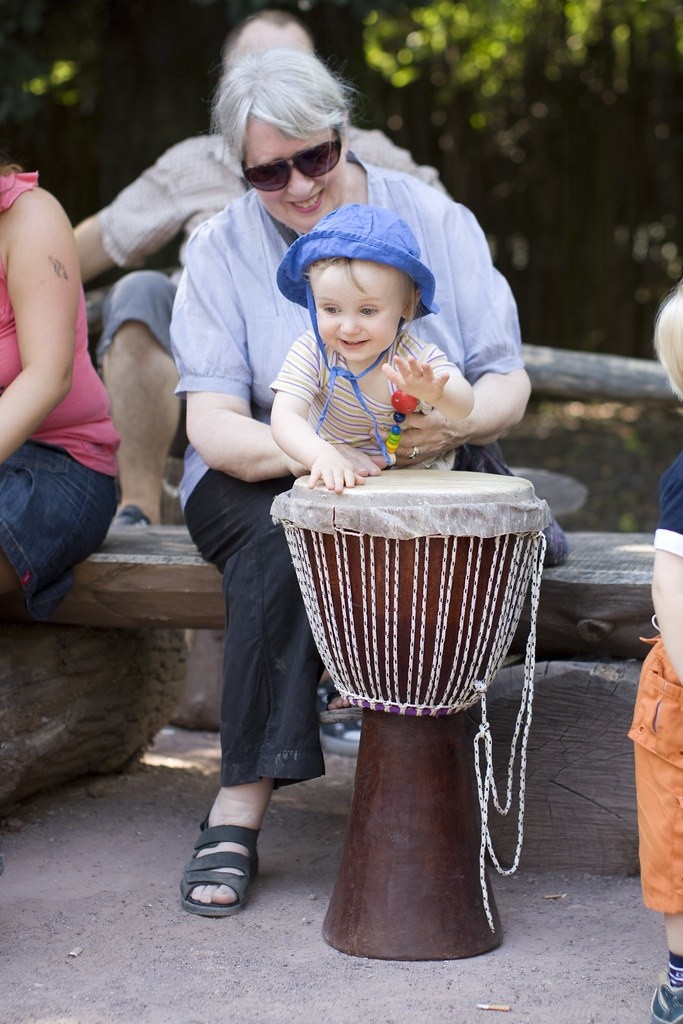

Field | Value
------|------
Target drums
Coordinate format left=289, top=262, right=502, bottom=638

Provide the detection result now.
left=292, top=469, right=537, bottom=965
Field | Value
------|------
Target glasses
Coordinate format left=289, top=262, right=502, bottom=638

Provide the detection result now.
left=240, top=126, right=343, bottom=191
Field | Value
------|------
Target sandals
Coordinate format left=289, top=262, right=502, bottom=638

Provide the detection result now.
left=316, top=682, right=366, bottom=724
left=179, top=811, right=261, bottom=917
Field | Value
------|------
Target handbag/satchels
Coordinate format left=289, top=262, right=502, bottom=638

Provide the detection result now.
left=461, top=439, right=570, bottom=568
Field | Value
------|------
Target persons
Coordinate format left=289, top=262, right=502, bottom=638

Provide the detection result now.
left=627, top=283, right=683, bottom=1024
left=270, top=205, right=473, bottom=494
left=171, top=54, right=532, bottom=915
left=0, top=11, right=449, bottom=620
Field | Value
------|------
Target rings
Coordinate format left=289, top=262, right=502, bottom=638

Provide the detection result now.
left=407, top=447, right=419, bottom=460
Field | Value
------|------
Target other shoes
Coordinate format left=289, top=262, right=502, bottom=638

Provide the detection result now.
left=650, top=970, right=683, bottom=1024
left=112, top=503, right=149, bottom=527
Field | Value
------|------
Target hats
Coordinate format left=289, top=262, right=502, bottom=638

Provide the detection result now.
left=277, top=202, right=442, bottom=321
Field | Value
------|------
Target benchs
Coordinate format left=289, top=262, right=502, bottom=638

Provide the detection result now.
left=0, top=522, right=657, bottom=662
